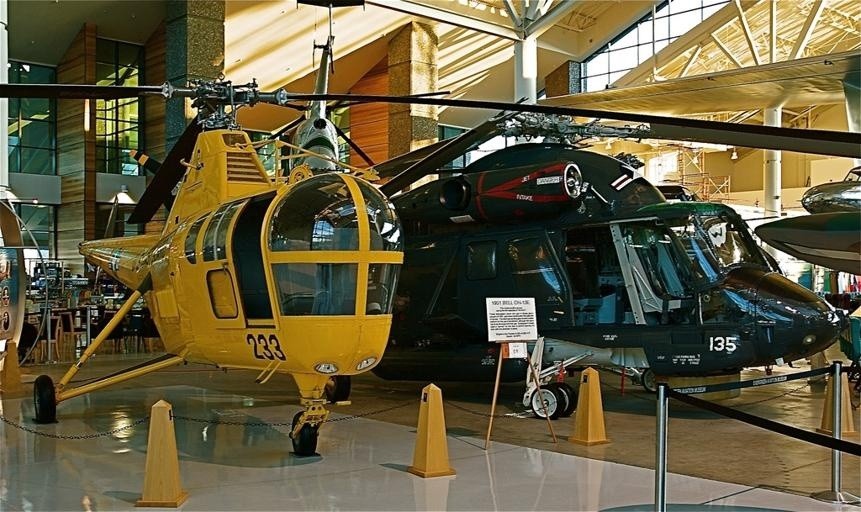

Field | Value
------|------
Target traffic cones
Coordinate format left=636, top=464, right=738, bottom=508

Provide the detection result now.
left=568, top=367, right=611, bottom=448
left=410, top=383, right=460, bottom=482
left=136, top=396, right=193, bottom=510
